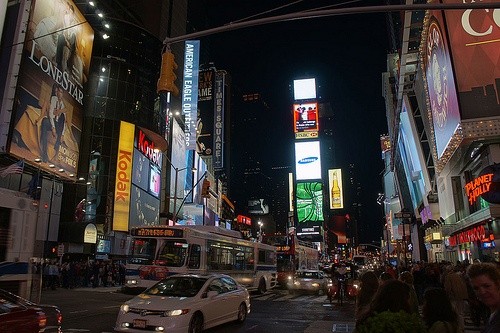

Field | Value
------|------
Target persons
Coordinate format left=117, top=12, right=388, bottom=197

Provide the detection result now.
left=37, top=82, right=68, bottom=163
left=329, top=253, right=500, bottom=333
left=32, top=0, right=77, bottom=78
left=29, top=259, right=125, bottom=289
left=295, top=106, right=317, bottom=132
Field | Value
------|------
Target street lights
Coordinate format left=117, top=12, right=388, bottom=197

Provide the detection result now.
left=153, top=148, right=197, bottom=226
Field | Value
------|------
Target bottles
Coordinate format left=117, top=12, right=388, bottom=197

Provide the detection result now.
left=332, top=172, right=341, bottom=207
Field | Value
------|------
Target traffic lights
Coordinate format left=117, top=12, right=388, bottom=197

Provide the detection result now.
left=46, top=241, right=59, bottom=257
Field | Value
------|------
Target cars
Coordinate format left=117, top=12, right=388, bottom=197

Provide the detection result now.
left=115, top=271, right=252, bottom=333
left=0, top=288, right=63, bottom=333
left=0, top=260, right=39, bottom=286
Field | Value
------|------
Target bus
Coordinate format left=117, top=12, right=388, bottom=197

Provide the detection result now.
left=120, top=225, right=279, bottom=295
left=259, top=232, right=382, bottom=306
